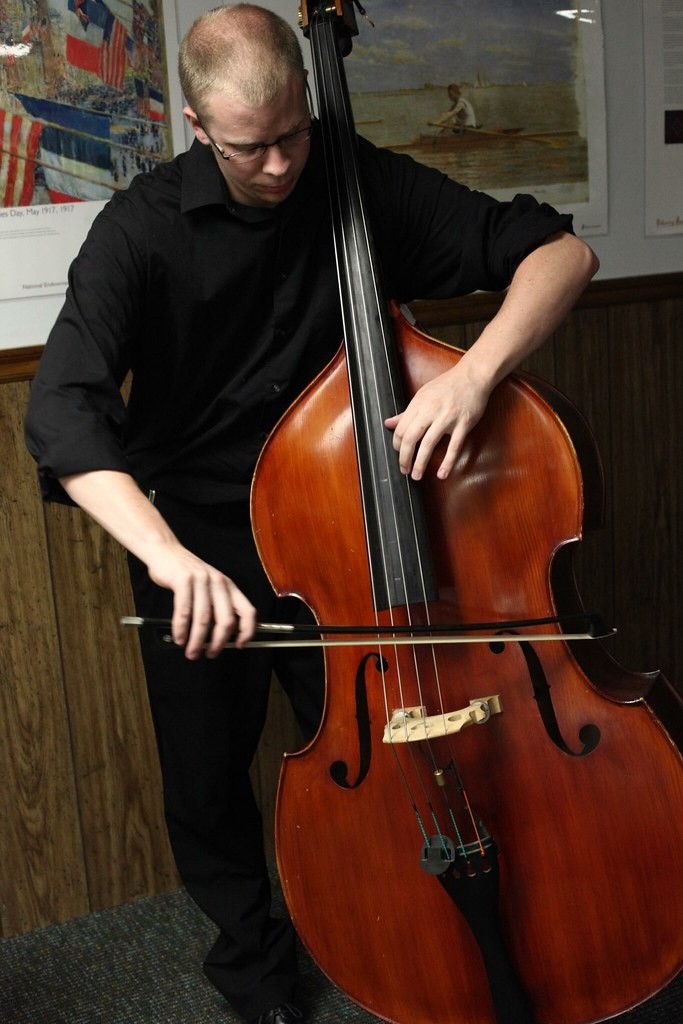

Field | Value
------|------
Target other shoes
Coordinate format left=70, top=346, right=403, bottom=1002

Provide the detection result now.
left=253, top=1001, right=303, bottom=1024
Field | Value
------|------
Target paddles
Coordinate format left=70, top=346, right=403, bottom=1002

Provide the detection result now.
left=426, top=118, right=570, bottom=150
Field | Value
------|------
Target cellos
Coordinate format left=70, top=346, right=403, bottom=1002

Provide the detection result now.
left=235, top=1, right=682, bottom=1024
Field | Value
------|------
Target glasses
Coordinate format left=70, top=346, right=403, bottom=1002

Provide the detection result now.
left=196, top=77, right=317, bottom=163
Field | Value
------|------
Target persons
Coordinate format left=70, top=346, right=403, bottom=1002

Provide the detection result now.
left=24, top=4, right=600, bottom=1024
